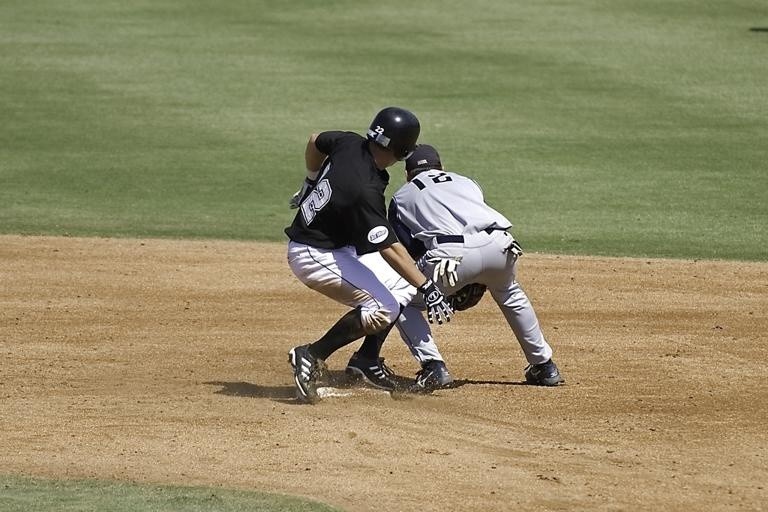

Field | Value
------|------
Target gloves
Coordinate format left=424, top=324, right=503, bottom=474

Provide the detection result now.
left=433, top=260, right=460, bottom=287
left=290, top=177, right=316, bottom=209
left=418, top=280, right=454, bottom=325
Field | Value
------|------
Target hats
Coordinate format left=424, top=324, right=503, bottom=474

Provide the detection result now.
left=406, top=144, right=441, bottom=172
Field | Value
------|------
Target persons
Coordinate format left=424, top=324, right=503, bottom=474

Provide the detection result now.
left=383, top=143, right=567, bottom=391
left=282, top=106, right=456, bottom=405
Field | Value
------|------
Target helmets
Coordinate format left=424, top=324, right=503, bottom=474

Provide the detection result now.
left=367, top=108, right=420, bottom=161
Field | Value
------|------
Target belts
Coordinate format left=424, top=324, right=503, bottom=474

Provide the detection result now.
left=437, top=228, right=496, bottom=244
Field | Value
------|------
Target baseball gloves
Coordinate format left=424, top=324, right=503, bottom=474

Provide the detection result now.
left=447, top=284, right=487, bottom=311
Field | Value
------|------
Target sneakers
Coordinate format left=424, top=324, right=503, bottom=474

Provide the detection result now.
left=288, top=344, right=328, bottom=405
left=409, top=360, right=453, bottom=393
left=346, top=353, right=398, bottom=390
left=525, top=359, right=564, bottom=386
